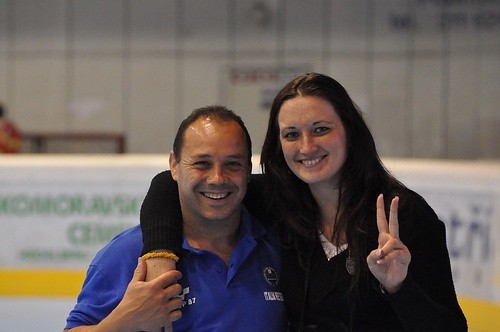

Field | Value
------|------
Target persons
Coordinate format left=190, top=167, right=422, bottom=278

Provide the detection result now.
left=64, top=104, right=294, bottom=332
left=139, top=72, right=468, bottom=332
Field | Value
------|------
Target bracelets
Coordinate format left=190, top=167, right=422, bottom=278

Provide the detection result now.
left=142, top=251, right=179, bottom=262
